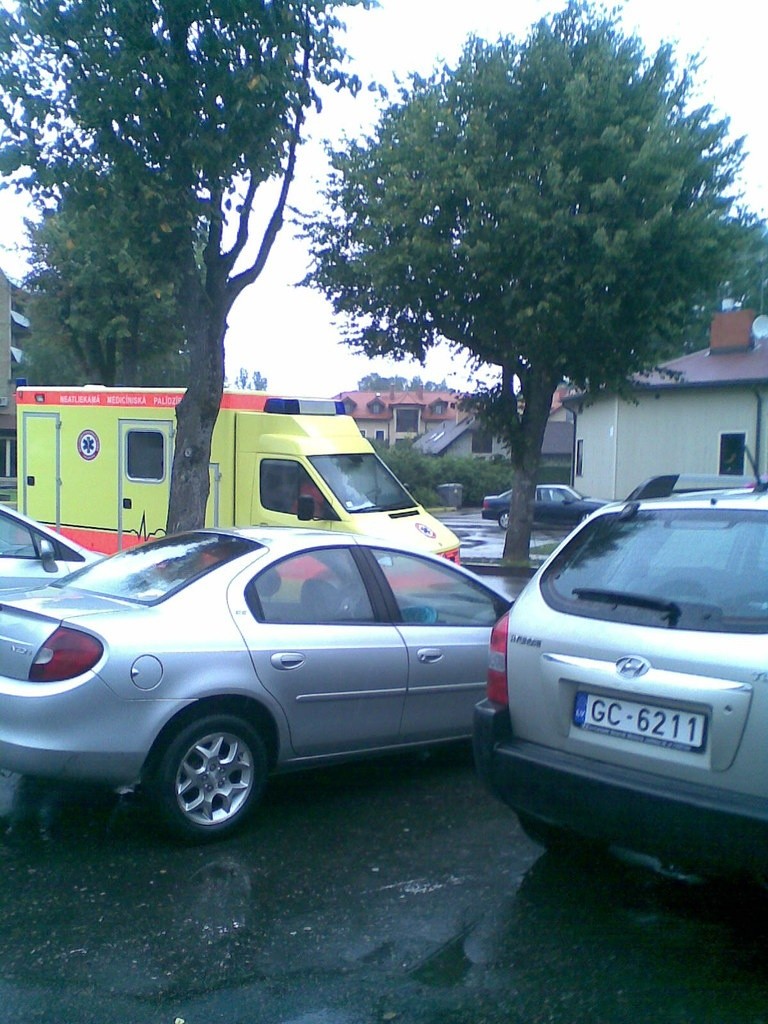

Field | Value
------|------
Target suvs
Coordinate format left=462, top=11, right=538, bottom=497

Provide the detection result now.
left=466, top=471, right=768, bottom=878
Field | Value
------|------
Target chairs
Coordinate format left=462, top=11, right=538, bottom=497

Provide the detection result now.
left=258, top=569, right=290, bottom=622
left=300, top=578, right=349, bottom=621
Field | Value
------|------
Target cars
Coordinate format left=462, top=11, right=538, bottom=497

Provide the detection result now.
left=0, top=526, right=517, bottom=849
left=0, top=506, right=113, bottom=589
left=480, top=484, right=618, bottom=528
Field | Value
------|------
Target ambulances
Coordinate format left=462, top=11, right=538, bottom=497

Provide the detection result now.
left=14, top=388, right=461, bottom=567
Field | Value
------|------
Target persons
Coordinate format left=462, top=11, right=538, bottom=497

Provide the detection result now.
left=291, top=480, right=323, bottom=517
